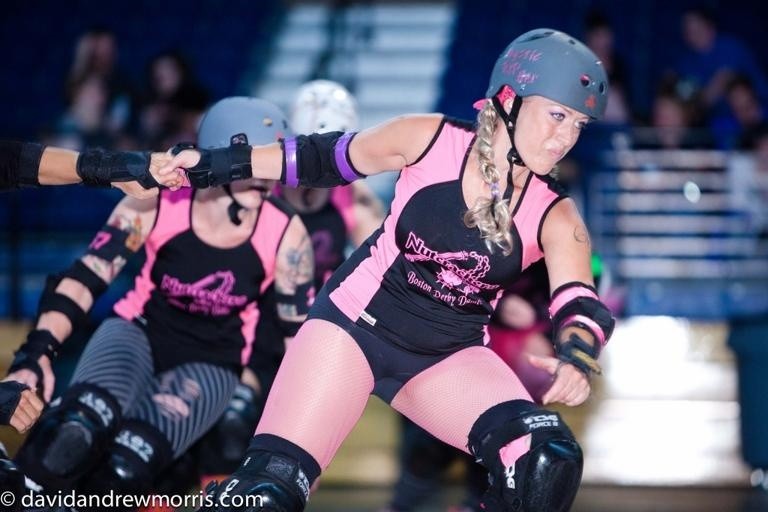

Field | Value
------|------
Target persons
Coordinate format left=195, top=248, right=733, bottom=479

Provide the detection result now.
left=0, top=0, right=768, bottom=512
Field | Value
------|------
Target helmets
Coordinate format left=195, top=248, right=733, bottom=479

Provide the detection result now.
left=484, top=27, right=609, bottom=123
left=285, top=79, right=358, bottom=136
left=196, top=96, right=296, bottom=152
